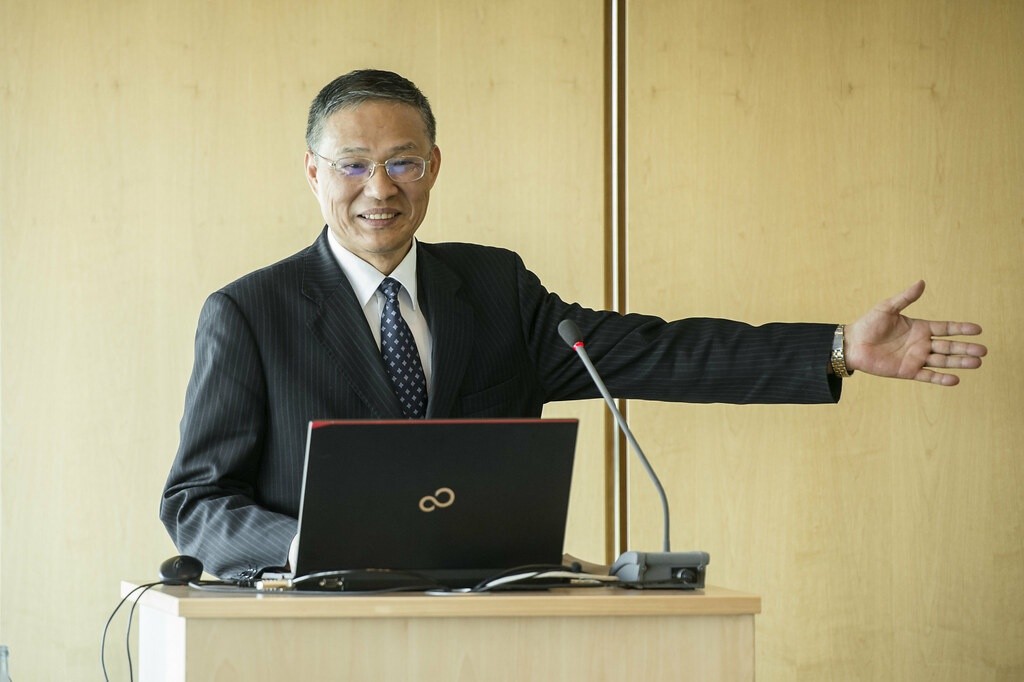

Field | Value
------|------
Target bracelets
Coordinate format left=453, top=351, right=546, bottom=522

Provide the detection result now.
left=831, top=324, right=854, bottom=377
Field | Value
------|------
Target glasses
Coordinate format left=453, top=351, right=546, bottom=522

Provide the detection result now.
left=313, top=151, right=431, bottom=183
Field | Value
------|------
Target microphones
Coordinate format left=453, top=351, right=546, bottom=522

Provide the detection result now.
left=557, top=318, right=710, bottom=592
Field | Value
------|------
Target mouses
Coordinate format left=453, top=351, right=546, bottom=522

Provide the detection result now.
left=158, top=554, right=204, bottom=586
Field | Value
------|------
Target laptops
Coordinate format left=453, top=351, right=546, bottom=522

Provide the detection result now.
left=256, top=417, right=578, bottom=594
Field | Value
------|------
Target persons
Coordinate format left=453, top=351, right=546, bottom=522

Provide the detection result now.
left=160, top=69, right=986, bottom=588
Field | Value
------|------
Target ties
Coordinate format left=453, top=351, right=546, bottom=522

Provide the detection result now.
left=375, top=278, right=428, bottom=419
